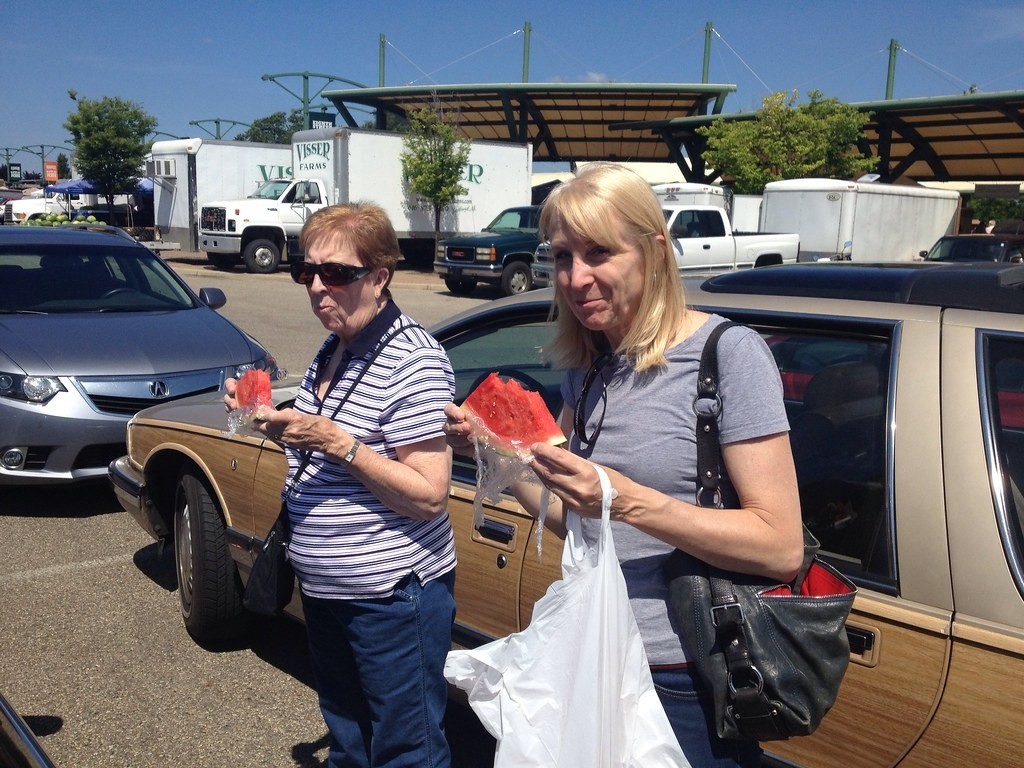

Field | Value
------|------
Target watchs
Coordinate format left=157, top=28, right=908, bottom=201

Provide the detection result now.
left=341, top=440, right=360, bottom=468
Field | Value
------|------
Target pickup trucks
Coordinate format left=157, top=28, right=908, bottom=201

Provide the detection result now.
left=532, top=206, right=800, bottom=288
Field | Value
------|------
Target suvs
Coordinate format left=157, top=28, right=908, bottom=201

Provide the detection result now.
left=0, top=223, right=280, bottom=487
left=433, top=205, right=549, bottom=296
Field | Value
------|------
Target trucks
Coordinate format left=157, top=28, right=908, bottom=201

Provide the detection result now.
left=3, top=156, right=153, bottom=224
left=759, top=177, right=961, bottom=264
left=198, top=126, right=533, bottom=275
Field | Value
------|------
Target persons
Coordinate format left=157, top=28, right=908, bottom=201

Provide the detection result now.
left=223, top=199, right=458, bottom=768
left=442, top=162, right=804, bottom=768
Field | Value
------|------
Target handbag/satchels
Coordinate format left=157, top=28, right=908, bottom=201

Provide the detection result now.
left=443, top=464, right=692, bottom=768
left=242, top=502, right=295, bottom=615
left=664, top=320, right=858, bottom=739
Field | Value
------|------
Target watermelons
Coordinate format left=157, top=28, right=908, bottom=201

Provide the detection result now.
left=20, top=213, right=106, bottom=227
left=235, top=369, right=272, bottom=421
left=460, top=372, right=567, bottom=456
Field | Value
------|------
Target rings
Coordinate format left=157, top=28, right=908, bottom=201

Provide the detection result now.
left=274, top=433, right=279, bottom=440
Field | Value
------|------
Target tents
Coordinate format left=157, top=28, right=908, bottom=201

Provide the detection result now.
left=44, top=175, right=155, bottom=227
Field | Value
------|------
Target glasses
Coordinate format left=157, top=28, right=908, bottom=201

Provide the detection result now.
left=290, top=261, right=371, bottom=286
left=573, top=353, right=614, bottom=445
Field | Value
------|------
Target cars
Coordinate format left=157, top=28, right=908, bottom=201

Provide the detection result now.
left=107, top=263, right=1024, bottom=768
left=918, top=232, right=1024, bottom=263
